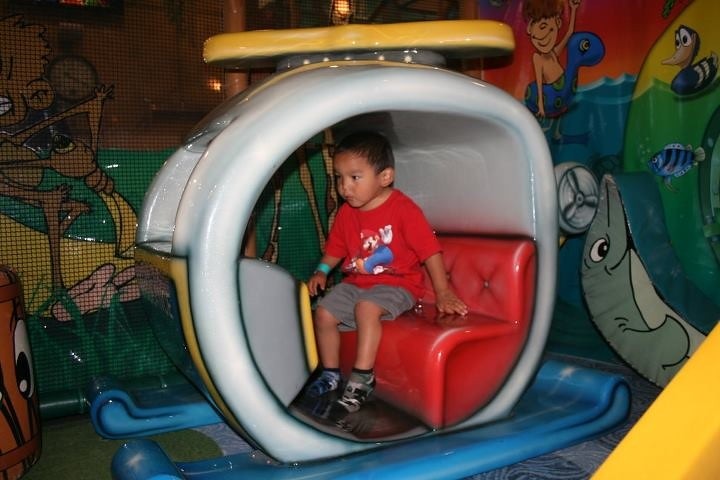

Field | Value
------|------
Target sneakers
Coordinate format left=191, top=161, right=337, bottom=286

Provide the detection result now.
left=334, top=373, right=376, bottom=413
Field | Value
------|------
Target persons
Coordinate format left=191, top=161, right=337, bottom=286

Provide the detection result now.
left=293, top=133, right=468, bottom=424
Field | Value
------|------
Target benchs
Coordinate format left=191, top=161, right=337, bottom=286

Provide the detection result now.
left=313, top=235, right=536, bottom=428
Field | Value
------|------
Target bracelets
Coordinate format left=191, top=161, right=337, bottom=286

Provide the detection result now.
left=313, top=263, right=331, bottom=275
